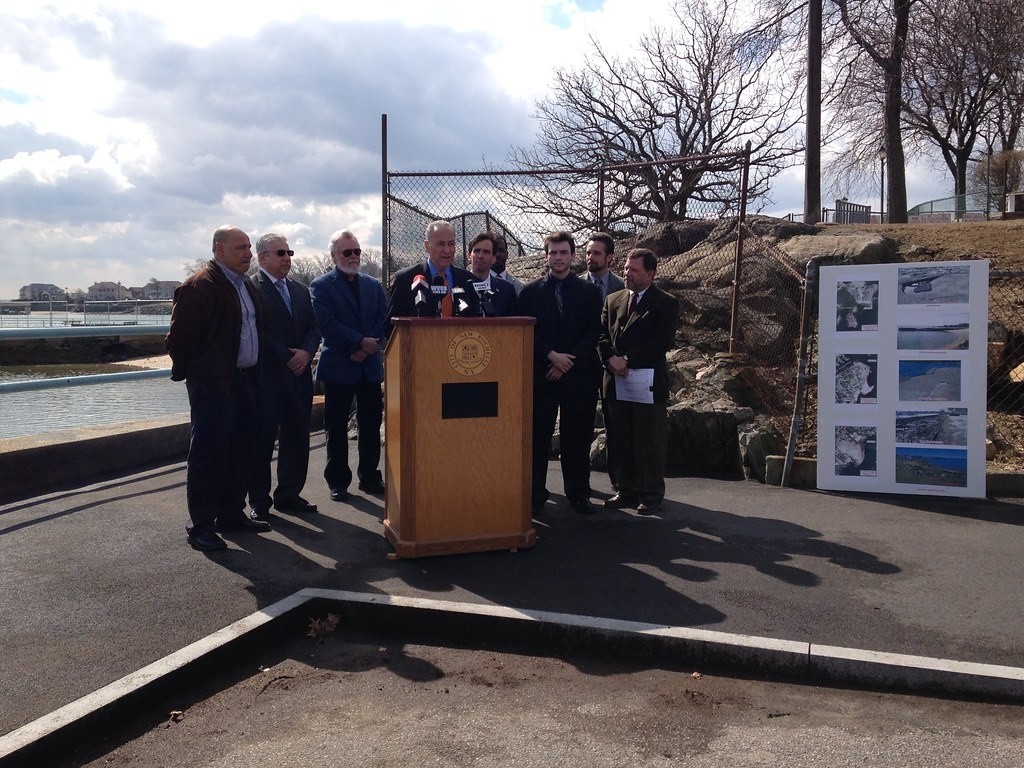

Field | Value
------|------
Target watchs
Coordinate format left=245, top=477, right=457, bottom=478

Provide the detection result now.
left=624, top=355, right=628, bottom=361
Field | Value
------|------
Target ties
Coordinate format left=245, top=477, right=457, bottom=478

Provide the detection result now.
left=496, top=274, right=502, bottom=278
left=596, top=279, right=603, bottom=300
left=628, top=293, right=638, bottom=319
left=438, top=271, right=453, bottom=317
left=555, top=281, right=563, bottom=319
left=275, top=281, right=294, bottom=318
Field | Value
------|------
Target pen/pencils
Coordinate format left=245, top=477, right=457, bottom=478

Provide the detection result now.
left=642, top=311, right=649, bottom=320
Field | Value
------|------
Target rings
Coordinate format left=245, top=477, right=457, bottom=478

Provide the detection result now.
left=297, top=365, right=300, bottom=367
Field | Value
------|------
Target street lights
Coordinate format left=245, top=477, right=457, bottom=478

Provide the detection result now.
left=877, top=145, right=887, bottom=223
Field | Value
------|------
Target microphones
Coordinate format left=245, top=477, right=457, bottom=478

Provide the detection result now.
left=431, top=275, right=447, bottom=314
left=450, top=283, right=471, bottom=315
left=411, top=275, right=429, bottom=307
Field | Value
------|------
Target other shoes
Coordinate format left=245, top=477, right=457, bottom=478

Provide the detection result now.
left=532, top=501, right=545, bottom=515
left=568, top=498, right=596, bottom=513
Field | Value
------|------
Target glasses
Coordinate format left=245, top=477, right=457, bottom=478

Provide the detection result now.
left=265, top=250, right=295, bottom=256
left=337, top=248, right=361, bottom=257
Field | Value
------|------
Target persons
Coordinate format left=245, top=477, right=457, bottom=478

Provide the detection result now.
left=595, top=248, right=679, bottom=513
left=467, top=231, right=518, bottom=315
left=491, top=234, right=525, bottom=296
left=168, top=226, right=269, bottom=552
left=249, top=234, right=318, bottom=519
left=384, top=221, right=482, bottom=342
left=578, top=234, right=625, bottom=489
left=309, top=229, right=386, bottom=500
left=516, top=231, right=604, bottom=513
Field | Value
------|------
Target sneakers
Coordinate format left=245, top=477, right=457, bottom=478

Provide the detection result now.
left=605, top=491, right=639, bottom=507
left=637, top=502, right=660, bottom=514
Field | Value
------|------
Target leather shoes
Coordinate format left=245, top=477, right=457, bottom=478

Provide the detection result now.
left=276, top=495, right=317, bottom=512
left=187, top=527, right=226, bottom=550
left=216, top=513, right=270, bottom=531
left=250, top=507, right=269, bottom=520
left=359, top=479, right=386, bottom=494
left=331, top=486, right=347, bottom=499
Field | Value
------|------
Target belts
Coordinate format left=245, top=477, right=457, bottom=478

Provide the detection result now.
left=236, top=367, right=249, bottom=374
